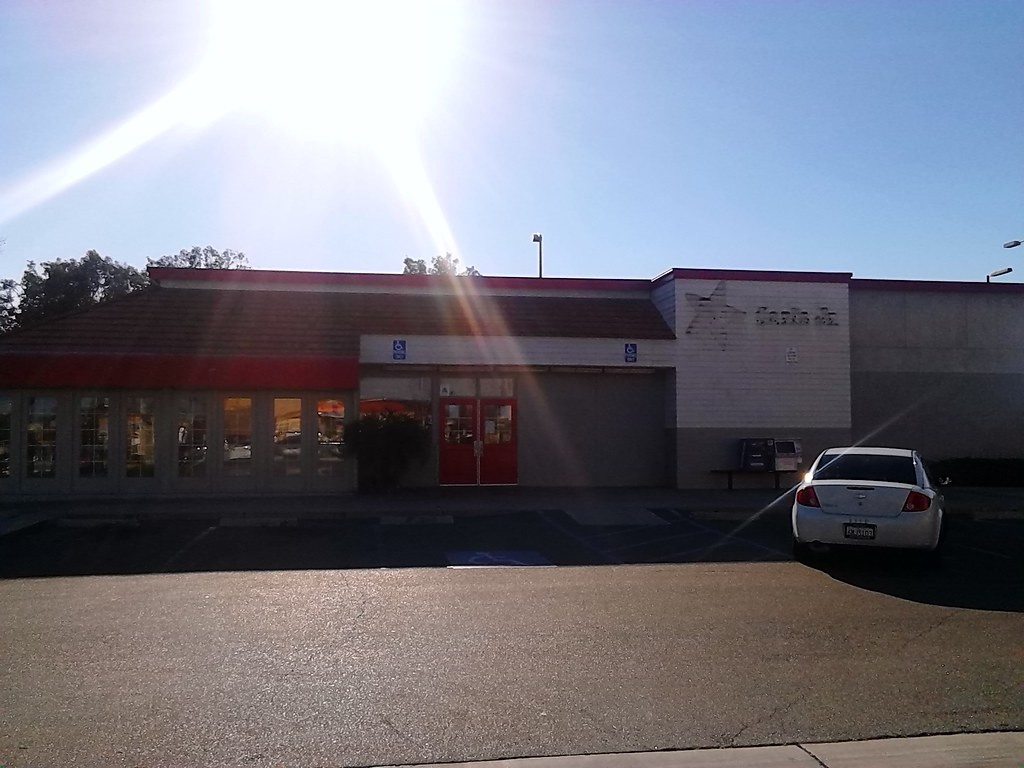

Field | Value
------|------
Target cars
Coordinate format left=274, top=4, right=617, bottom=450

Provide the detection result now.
left=178, top=444, right=206, bottom=460
left=28, top=456, right=54, bottom=474
left=793, top=446, right=954, bottom=554
left=226, top=443, right=252, bottom=459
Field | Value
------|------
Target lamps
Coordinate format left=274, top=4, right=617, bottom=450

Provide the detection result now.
left=986, top=268, right=1013, bottom=283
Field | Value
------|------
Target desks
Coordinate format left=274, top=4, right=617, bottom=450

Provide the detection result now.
left=711, top=469, right=798, bottom=489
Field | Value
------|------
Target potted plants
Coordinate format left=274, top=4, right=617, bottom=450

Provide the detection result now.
left=333, top=409, right=434, bottom=496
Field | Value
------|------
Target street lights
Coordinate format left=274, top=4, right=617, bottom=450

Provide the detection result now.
left=532, top=233, right=542, bottom=277
